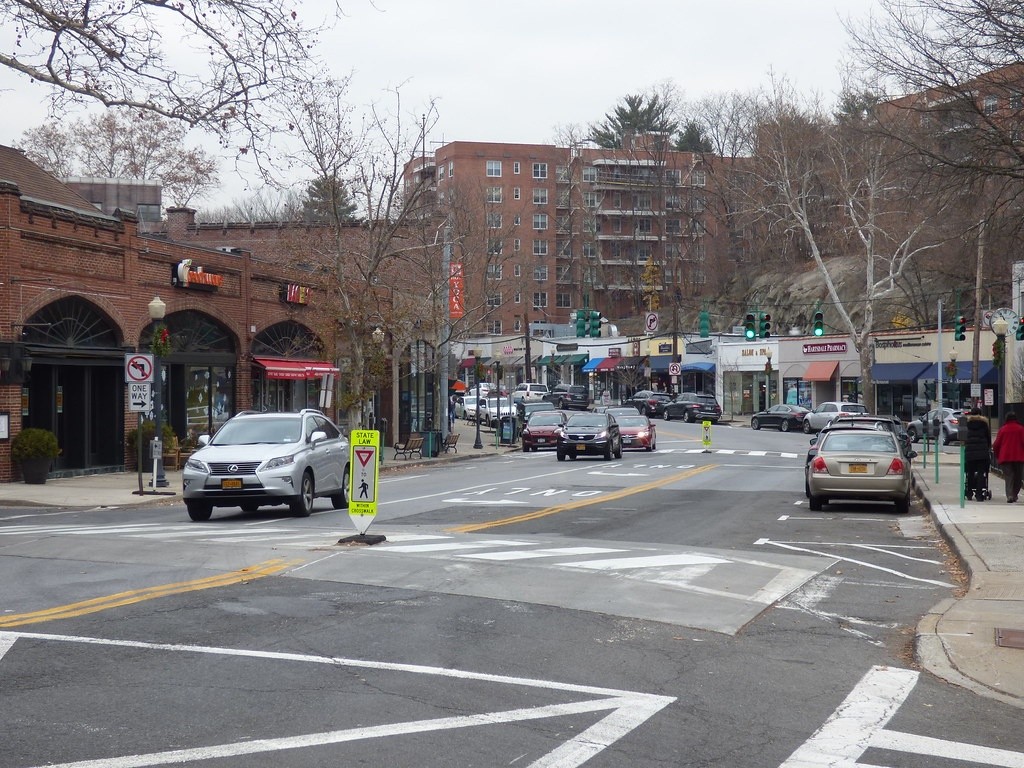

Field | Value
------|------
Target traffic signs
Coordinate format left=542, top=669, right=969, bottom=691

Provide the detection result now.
left=128, top=382, right=151, bottom=413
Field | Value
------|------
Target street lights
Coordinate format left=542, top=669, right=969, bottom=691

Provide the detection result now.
left=948, top=346, right=958, bottom=409
left=473, top=345, right=484, bottom=449
left=371, top=327, right=384, bottom=461
left=765, top=347, right=773, bottom=409
left=149, top=297, right=169, bottom=487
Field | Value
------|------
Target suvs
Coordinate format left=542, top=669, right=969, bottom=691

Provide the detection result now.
left=663, top=392, right=722, bottom=425
left=542, top=383, right=590, bottom=411
left=906, top=407, right=971, bottom=445
left=803, top=402, right=869, bottom=434
left=507, top=382, right=549, bottom=404
left=182, top=408, right=351, bottom=522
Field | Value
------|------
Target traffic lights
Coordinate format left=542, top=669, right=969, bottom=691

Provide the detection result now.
left=759, top=312, right=770, bottom=338
left=576, top=309, right=586, bottom=337
left=955, top=315, right=966, bottom=341
left=1016, top=316, right=1024, bottom=340
left=745, top=313, right=756, bottom=341
left=486, top=368, right=491, bottom=374
left=588, top=309, right=601, bottom=338
left=813, top=310, right=824, bottom=336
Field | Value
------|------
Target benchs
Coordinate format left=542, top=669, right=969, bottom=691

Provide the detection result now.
left=442, top=433, right=461, bottom=454
left=393, top=437, right=424, bottom=460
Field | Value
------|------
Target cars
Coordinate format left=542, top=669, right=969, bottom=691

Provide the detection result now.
left=455, top=382, right=556, bottom=437
left=751, top=404, right=811, bottom=432
left=625, top=389, right=676, bottom=418
left=522, top=411, right=568, bottom=452
left=557, top=412, right=623, bottom=461
left=805, top=415, right=918, bottom=513
left=591, top=405, right=657, bottom=452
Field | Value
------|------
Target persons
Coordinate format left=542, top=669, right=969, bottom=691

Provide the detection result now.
left=993, top=412, right=1024, bottom=503
left=964, top=408, right=992, bottom=502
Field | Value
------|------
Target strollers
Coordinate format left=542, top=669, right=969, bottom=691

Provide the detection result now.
left=964, top=449, right=992, bottom=500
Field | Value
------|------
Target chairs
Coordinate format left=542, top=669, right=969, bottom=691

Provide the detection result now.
left=832, top=444, right=849, bottom=450
left=871, top=445, right=889, bottom=452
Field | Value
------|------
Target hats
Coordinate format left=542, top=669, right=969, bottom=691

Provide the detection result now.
left=1004, top=411, right=1017, bottom=421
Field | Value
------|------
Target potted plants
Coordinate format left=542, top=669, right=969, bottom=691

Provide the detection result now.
left=11, top=428, right=62, bottom=486
left=128, top=419, right=197, bottom=473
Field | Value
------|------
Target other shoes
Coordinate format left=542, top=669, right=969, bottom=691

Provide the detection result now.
left=1007, top=496, right=1017, bottom=503
left=976, top=495, right=984, bottom=502
left=966, top=495, right=973, bottom=499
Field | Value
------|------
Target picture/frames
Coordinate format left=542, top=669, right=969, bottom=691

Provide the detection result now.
left=0, top=411, right=11, bottom=441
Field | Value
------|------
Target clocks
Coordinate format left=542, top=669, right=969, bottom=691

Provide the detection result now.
left=989, top=307, right=1020, bottom=338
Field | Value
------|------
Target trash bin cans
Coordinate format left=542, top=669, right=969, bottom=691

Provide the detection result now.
left=502, top=416, right=517, bottom=444
left=420, top=431, right=439, bottom=457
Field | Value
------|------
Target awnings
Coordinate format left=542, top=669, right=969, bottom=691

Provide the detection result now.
left=255, top=358, right=339, bottom=380
left=461, top=355, right=542, bottom=369
left=583, top=355, right=681, bottom=373
left=920, top=360, right=998, bottom=383
left=855, top=362, right=933, bottom=384
left=681, top=362, right=715, bottom=373
left=537, top=354, right=589, bottom=366
left=803, top=361, right=838, bottom=381
